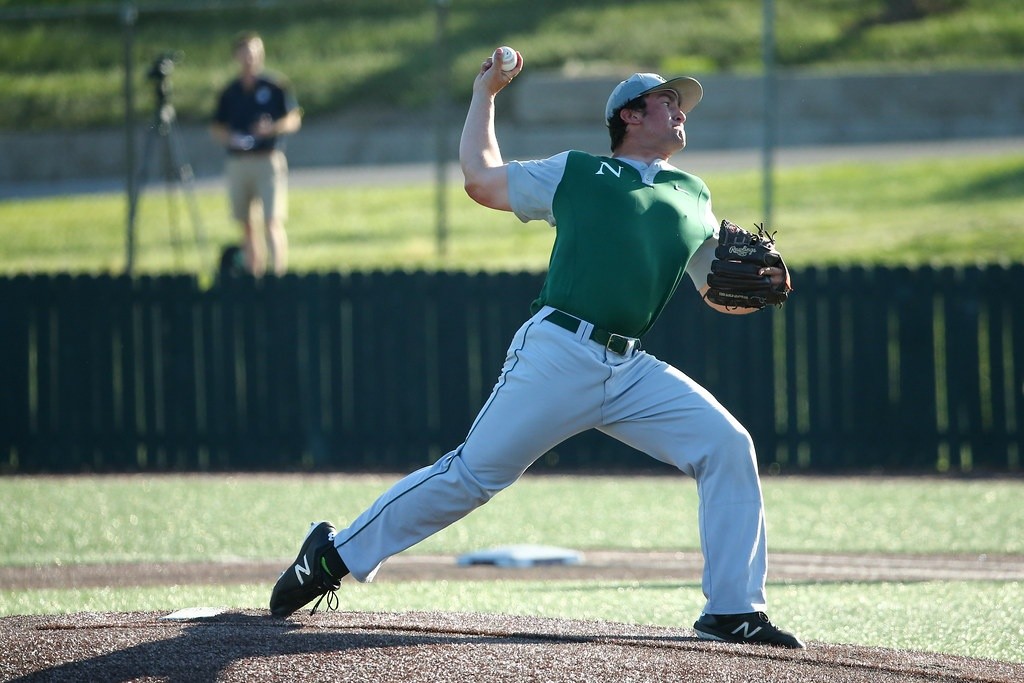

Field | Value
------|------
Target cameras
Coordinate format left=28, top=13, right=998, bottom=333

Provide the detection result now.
left=148, top=55, right=175, bottom=82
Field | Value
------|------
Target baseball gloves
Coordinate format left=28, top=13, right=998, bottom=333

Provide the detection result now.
left=706, top=219, right=791, bottom=309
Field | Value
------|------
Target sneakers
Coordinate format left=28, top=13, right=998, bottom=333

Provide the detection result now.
left=693, top=611, right=806, bottom=649
left=270, top=519, right=337, bottom=618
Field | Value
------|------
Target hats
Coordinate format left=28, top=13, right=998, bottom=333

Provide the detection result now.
left=605, top=73, right=702, bottom=128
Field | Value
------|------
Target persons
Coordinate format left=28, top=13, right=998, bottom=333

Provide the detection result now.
left=211, top=30, right=302, bottom=280
left=270, top=48, right=806, bottom=650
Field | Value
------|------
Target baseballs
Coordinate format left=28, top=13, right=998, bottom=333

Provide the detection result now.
left=492, top=46, right=518, bottom=72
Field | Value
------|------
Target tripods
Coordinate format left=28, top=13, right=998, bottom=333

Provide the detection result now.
left=132, top=82, right=212, bottom=276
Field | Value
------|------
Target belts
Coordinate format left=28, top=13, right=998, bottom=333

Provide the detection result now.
left=544, top=310, right=639, bottom=357
